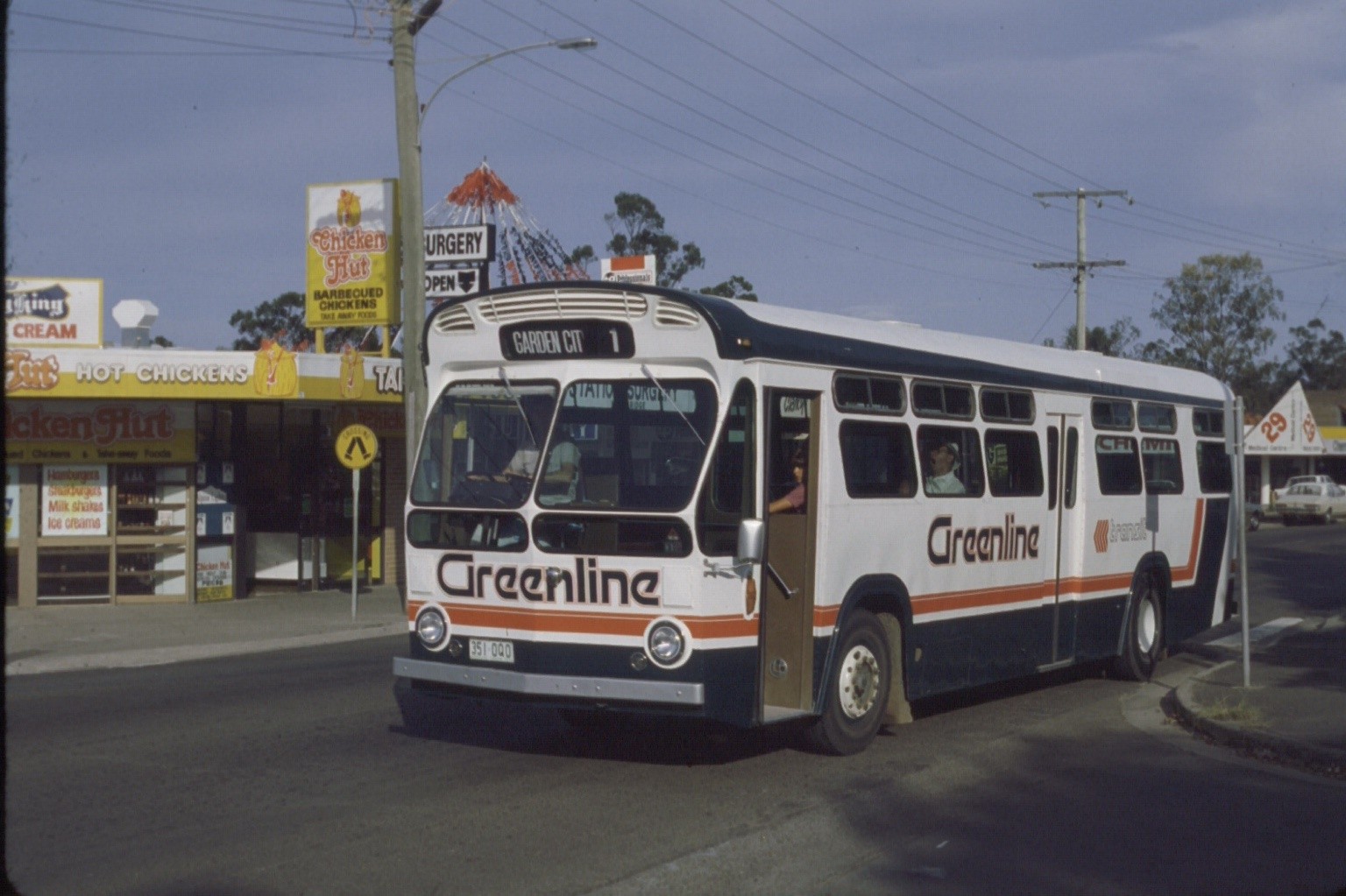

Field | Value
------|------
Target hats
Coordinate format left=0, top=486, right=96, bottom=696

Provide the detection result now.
left=938, top=437, right=960, bottom=464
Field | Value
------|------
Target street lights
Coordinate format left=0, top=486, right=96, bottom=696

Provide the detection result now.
left=398, top=37, right=598, bottom=484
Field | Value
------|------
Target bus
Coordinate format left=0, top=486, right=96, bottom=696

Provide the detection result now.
left=390, top=279, right=1238, bottom=754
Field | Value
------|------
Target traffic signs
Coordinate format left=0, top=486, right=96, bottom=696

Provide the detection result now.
left=424, top=223, right=497, bottom=301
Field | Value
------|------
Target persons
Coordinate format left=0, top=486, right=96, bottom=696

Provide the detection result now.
left=923, top=436, right=966, bottom=495
left=466, top=401, right=580, bottom=550
left=769, top=447, right=808, bottom=513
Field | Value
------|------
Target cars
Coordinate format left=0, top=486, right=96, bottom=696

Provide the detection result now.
left=1245, top=502, right=1264, bottom=531
left=1275, top=474, right=1336, bottom=499
left=1275, top=481, right=1346, bottom=525
